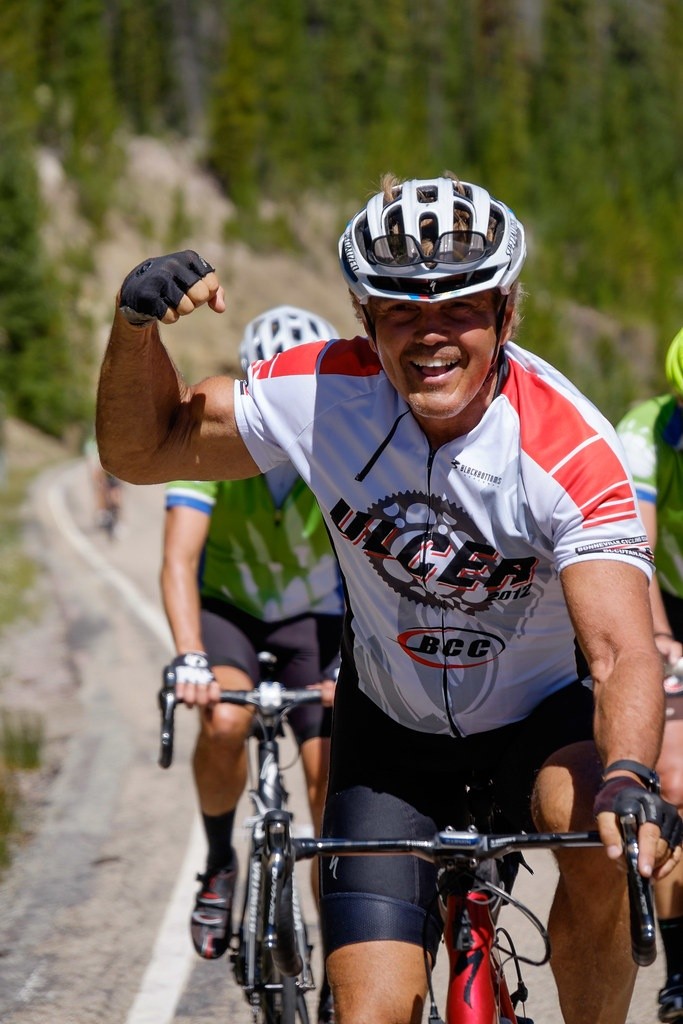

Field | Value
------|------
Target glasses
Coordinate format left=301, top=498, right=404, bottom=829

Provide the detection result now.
left=368, top=229, right=492, bottom=268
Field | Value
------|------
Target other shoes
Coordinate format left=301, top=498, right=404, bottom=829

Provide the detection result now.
left=190, top=846, right=239, bottom=961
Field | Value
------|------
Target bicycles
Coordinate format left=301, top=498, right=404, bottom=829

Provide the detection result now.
left=299, top=819, right=660, bottom=1023
left=151, top=670, right=333, bottom=1019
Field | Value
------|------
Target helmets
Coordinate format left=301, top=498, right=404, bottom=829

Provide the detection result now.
left=663, top=328, right=683, bottom=405
left=336, top=177, right=528, bottom=307
left=238, top=305, right=339, bottom=373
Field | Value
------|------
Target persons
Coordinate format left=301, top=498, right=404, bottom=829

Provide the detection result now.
left=90, top=175, right=681, bottom=1024
left=619, top=330, right=682, bottom=1024
left=156, top=302, right=348, bottom=961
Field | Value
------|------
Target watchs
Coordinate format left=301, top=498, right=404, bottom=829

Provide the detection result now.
left=602, top=759, right=665, bottom=798
